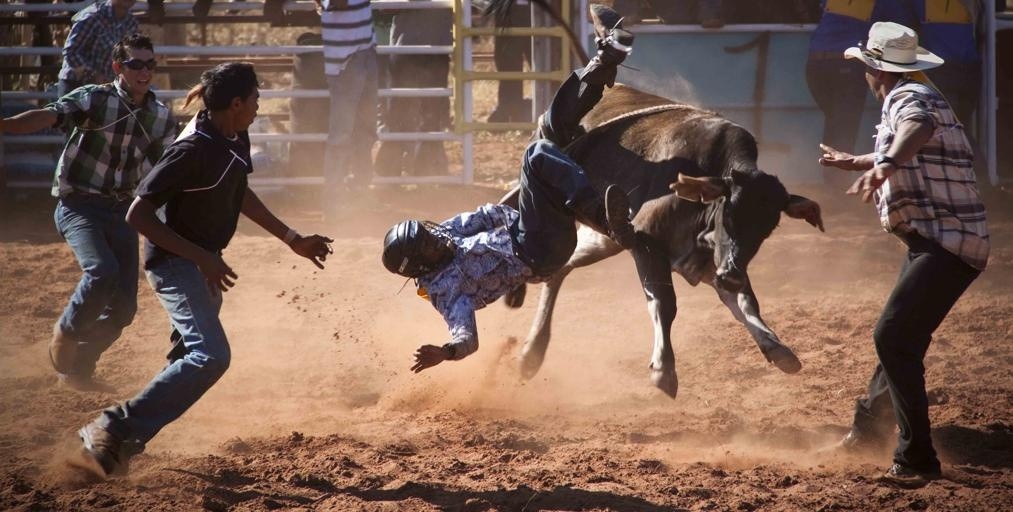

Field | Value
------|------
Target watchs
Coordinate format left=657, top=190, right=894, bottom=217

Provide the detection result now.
left=444, top=341, right=458, bottom=362
left=872, top=151, right=898, bottom=168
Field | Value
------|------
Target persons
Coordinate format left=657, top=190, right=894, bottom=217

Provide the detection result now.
left=1, top=34, right=188, bottom=392
left=371, top=6, right=455, bottom=198
left=76, top=62, right=335, bottom=475
left=54, top=0, right=140, bottom=141
left=381, top=3, right=640, bottom=374
left=313, top=1, right=379, bottom=240
left=818, top=18, right=990, bottom=489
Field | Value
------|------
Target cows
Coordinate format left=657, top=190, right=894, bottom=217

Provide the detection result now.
left=481, top=0, right=824, bottom=399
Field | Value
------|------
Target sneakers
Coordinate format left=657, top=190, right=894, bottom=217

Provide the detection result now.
left=880, top=460, right=931, bottom=487
left=812, top=431, right=893, bottom=463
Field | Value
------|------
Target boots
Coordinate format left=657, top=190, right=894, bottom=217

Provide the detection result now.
left=567, top=183, right=642, bottom=237
left=58, top=338, right=101, bottom=389
left=579, top=4, right=635, bottom=89
left=78, top=418, right=132, bottom=476
left=47, top=315, right=79, bottom=374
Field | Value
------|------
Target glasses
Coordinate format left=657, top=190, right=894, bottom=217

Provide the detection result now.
left=119, top=57, right=158, bottom=71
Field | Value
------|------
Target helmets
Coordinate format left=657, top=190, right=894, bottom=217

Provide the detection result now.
left=382, top=220, right=459, bottom=279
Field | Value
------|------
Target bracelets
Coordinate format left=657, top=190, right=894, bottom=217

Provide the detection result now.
left=282, top=225, right=299, bottom=246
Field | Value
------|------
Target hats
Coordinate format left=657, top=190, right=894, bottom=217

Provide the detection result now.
left=844, top=20, right=946, bottom=73
